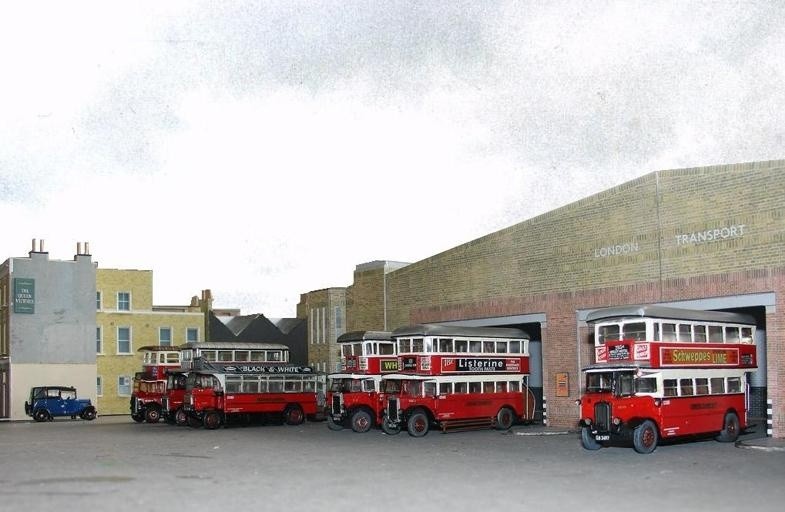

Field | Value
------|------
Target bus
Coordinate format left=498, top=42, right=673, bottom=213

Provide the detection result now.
left=164, top=342, right=291, bottom=428
left=375, top=322, right=530, bottom=436
left=183, top=358, right=320, bottom=430
left=324, top=330, right=398, bottom=434
left=128, top=345, right=184, bottom=425
left=575, top=305, right=757, bottom=454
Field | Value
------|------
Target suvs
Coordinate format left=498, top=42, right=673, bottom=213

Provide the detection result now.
left=24, top=385, right=97, bottom=423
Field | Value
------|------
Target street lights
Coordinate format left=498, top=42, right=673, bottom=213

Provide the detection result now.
left=383, top=262, right=390, bottom=330
